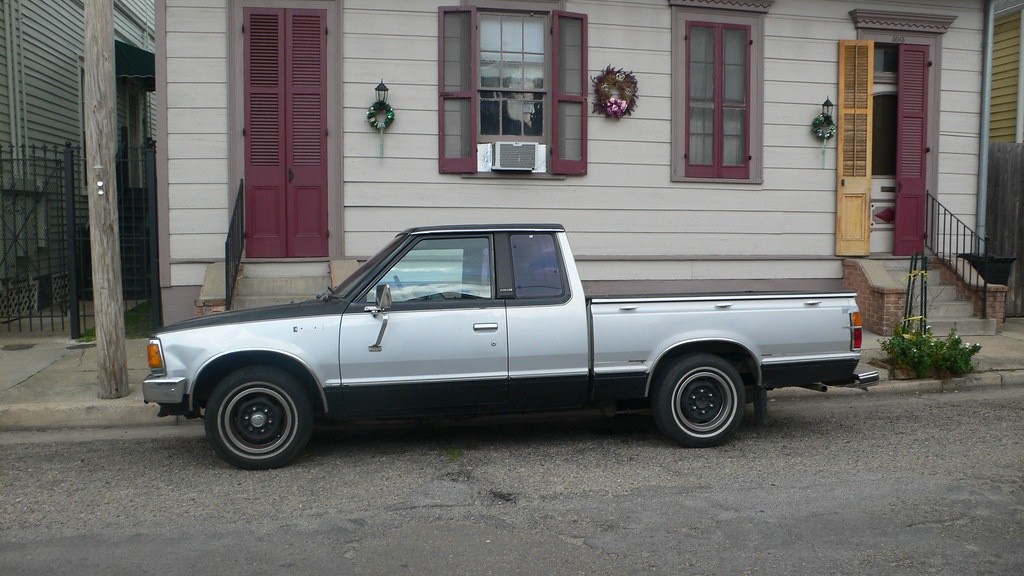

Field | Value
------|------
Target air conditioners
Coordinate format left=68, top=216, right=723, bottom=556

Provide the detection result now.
left=490, top=142, right=538, bottom=170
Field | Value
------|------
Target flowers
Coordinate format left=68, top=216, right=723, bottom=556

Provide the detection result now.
left=365, top=102, right=395, bottom=129
left=589, top=63, right=640, bottom=121
left=810, top=113, right=836, bottom=140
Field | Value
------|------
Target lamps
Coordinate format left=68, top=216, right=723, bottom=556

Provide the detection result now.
left=375, top=79, right=389, bottom=111
left=820, top=95, right=834, bottom=125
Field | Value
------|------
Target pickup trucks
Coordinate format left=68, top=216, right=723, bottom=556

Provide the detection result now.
left=142, top=222, right=879, bottom=473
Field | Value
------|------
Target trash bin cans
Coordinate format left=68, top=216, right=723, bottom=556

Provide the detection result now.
left=957, top=253, right=1018, bottom=323
